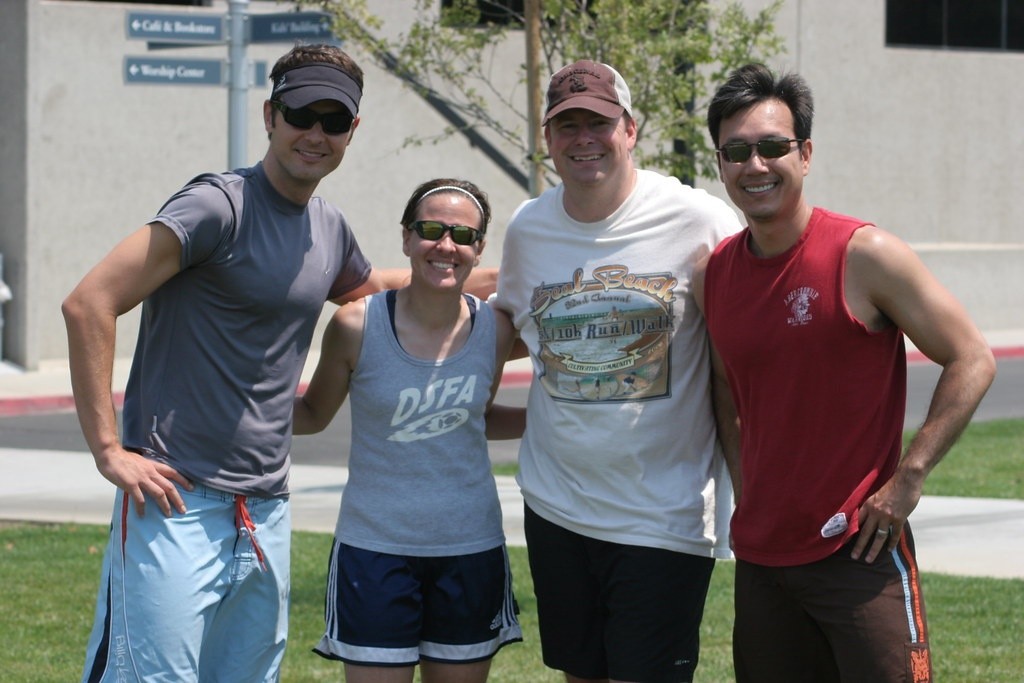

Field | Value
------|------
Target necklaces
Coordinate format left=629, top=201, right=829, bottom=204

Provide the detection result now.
left=61, top=44, right=502, bottom=683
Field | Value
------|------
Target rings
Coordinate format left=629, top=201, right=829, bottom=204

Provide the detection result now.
left=877, top=528, right=889, bottom=534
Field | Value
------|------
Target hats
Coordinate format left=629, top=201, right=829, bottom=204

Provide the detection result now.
left=272, top=62, right=362, bottom=119
left=539, top=58, right=633, bottom=127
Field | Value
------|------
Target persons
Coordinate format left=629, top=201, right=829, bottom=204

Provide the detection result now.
left=347, top=59, right=746, bottom=683
left=293, top=178, right=532, bottom=683
left=690, top=61, right=997, bottom=683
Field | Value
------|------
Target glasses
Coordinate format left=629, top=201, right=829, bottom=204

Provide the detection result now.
left=272, top=100, right=353, bottom=135
left=407, top=220, right=482, bottom=245
left=716, top=136, right=805, bottom=163
